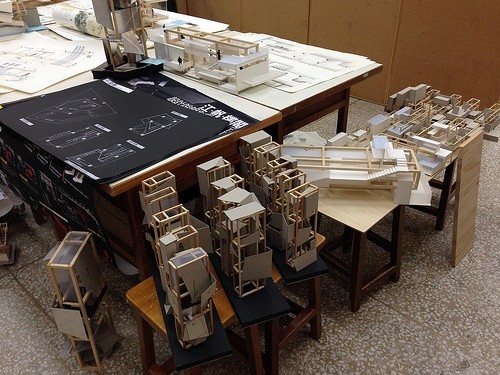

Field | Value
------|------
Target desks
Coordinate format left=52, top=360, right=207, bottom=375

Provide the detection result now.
left=0, top=0, right=383, bottom=283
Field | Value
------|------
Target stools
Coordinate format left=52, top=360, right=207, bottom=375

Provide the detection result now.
left=126, top=154, right=458, bottom=374
